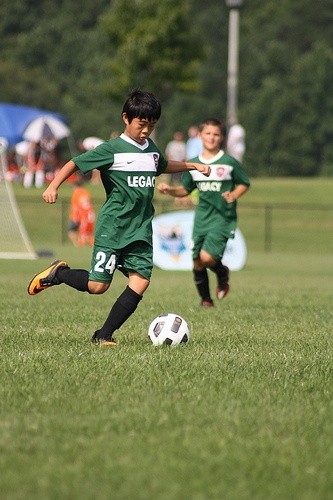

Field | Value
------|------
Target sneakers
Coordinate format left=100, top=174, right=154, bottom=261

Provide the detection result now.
left=92, top=328, right=122, bottom=347
left=28, top=259, right=71, bottom=296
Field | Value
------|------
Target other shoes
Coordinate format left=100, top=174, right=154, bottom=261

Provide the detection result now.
left=215, top=266, right=231, bottom=300
left=201, top=300, right=214, bottom=308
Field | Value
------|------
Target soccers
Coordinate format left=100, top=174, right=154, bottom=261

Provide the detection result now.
left=146, top=313, right=190, bottom=346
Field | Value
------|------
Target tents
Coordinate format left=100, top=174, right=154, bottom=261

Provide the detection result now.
left=0, top=102, right=77, bottom=162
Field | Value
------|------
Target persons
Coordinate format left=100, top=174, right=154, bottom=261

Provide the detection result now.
left=28, top=91, right=211, bottom=344
left=156, top=118, right=251, bottom=309
left=0, top=123, right=102, bottom=188
left=226, top=114, right=246, bottom=165
left=164, top=130, right=186, bottom=185
left=186, top=122, right=205, bottom=161
